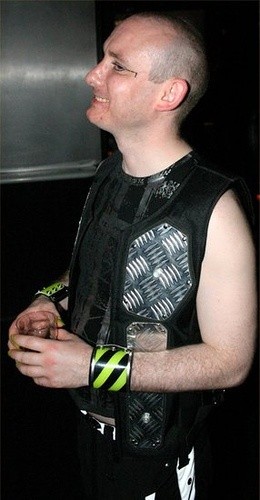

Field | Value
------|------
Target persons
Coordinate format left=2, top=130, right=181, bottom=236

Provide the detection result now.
left=8, top=8, right=256, bottom=500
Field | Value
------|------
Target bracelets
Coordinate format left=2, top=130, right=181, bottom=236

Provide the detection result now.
left=33, top=281, right=68, bottom=317
left=88, top=344, right=134, bottom=395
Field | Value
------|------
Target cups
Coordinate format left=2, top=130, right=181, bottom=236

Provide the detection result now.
left=16, top=311, right=58, bottom=351
